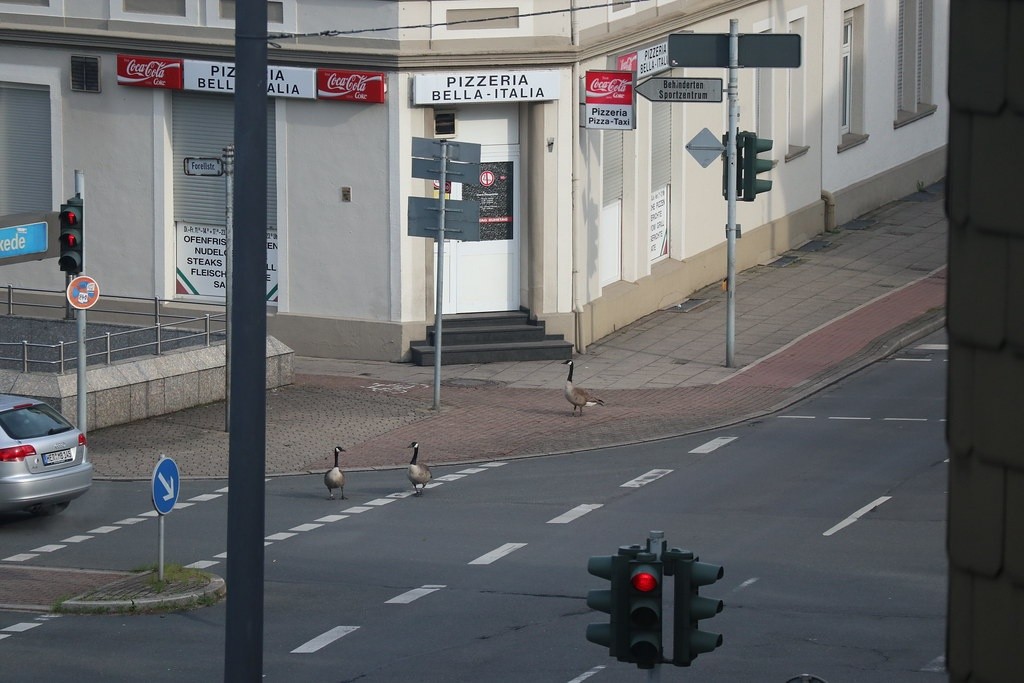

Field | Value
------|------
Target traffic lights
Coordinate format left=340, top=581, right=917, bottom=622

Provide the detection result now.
left=584, top=545, right=620, bottom=657
left=612, top=555, right=664, bottom=669
left=58, top=199, right=84, bottom=278
left=665, top=550, right=725, bottom=670
left=737, top=130, right=775, bottom=204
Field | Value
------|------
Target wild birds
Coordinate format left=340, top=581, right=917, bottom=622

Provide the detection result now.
left=560, top=359, right=605, bottom=417
left=407, top=441, right=433, bottom=497
left=323, top=446, right=349, bottom=500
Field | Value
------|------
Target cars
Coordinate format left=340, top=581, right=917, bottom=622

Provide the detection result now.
left=0, top=396, right=94, bottom=515
